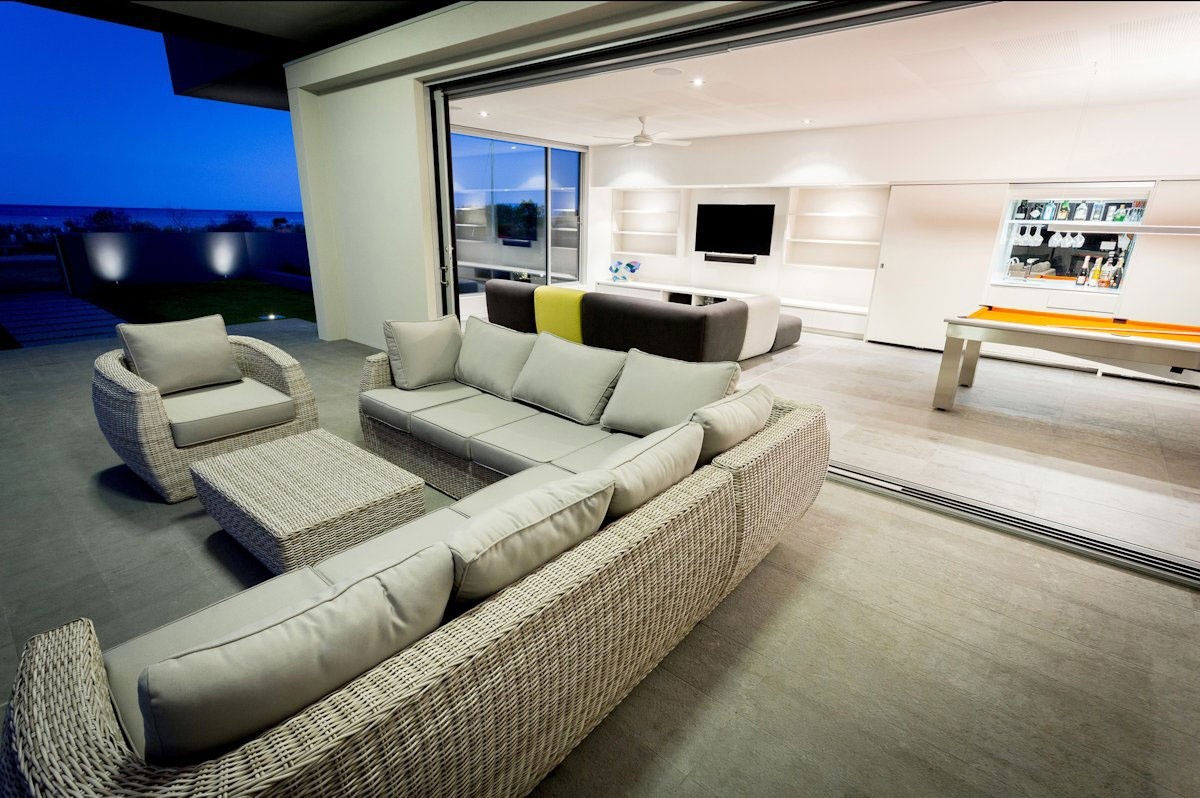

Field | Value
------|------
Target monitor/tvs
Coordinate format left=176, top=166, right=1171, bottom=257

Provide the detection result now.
left=696, top=204, right=776, bottom=256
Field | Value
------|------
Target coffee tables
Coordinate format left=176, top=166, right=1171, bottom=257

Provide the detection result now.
left=186, top=425, right=430, bottom=577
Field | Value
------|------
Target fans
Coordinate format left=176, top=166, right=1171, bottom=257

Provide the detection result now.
left=592, top=112, right=694, bottom=154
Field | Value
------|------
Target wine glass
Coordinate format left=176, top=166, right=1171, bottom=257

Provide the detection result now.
left=1123, top=207, right=1145, bottom=221
left=1072, top=232, right=1085, bottom=248
left=1048, top=232, right=1063, bottom=248
left=1013, top=225, right=1022, bottom=246
left=1020, top=225, right=1034, bottom=246
left=1033, top=225, right=1045, bottom=246
left=1061, top=232, right=1072, bottom=248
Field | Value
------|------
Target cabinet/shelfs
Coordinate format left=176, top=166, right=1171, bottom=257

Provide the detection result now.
left=603, top=178, right=898, bottom=339
left=987, top=192, right=1152, bottom=296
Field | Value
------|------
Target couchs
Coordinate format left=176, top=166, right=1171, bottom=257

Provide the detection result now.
left=87, top=309, right=322, bottom=505
left=701, top=291, right=804, bottom=363
left=0, top=310, right=737, bottom=798
left=354, top=312, right=834, bottom=620
left=482, top=275, right=705, bottom=364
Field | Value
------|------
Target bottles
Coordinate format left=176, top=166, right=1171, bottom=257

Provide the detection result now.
left=1088, top=257, right=1125, bottom=289
left=1015, top=200, right=1126, bottom=221
left=1076, top=255, right=1090, bottom=286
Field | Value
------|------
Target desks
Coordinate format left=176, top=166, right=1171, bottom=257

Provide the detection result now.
left=927, top=302, right=1200, bottom=415
